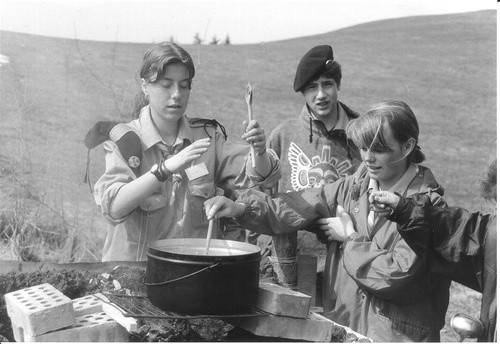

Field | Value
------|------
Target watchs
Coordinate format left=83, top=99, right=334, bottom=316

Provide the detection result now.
left=150, top=162, right=166, bottom=181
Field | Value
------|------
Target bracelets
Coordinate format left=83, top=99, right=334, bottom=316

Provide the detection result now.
left=160, top=159, right=172, bottom=177
left=252, top=146, right=267, bottom=155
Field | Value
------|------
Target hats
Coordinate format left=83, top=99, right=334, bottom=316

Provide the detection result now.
left=293, top=44, right=335, bottom=93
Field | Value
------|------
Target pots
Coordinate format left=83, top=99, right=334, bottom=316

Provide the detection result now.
left=145, top=238, right=262, bottom=316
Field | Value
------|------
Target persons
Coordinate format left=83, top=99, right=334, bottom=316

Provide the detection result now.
left=94, top=43, right=284, bottom=263
left=268, top=44, right=364, bottom=308
left=367, top=157, right=497, bottom=343
left=203, top=99, right=453, bottom=343
